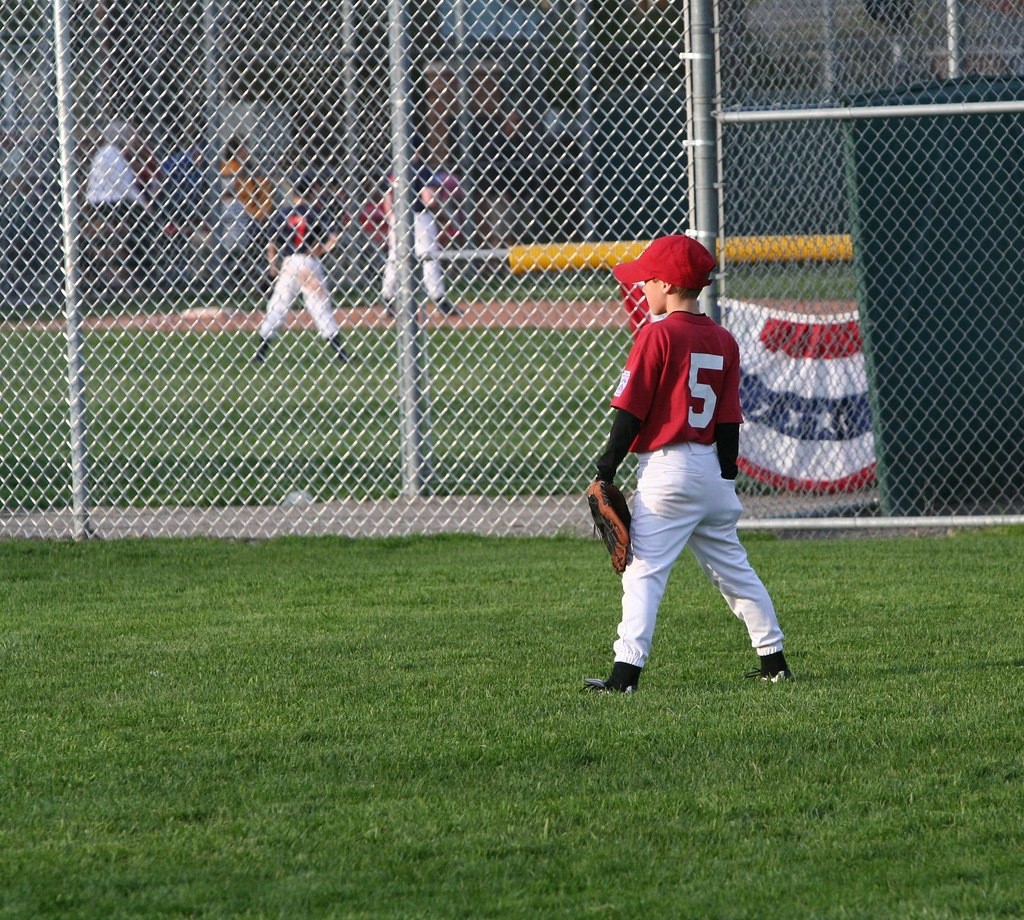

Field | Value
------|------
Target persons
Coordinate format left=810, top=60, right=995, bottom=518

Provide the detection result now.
left=381, top=136, right=464, bottom=319
left=77, top=116, right=279, bottom=261
left=250, top=174, right=363, bottom=365
left=578, top=234, right=796, bottom=695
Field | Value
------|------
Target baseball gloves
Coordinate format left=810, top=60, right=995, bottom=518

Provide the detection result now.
left=584, top=480, right=632, bottom=579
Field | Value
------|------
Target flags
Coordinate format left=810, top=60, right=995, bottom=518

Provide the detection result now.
left=715, top=296, right=876, bottom=498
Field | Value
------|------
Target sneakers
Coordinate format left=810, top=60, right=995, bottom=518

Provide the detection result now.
left=744, top=667, right=793, bottom=682
left=579, top=678, right=633, bottom=695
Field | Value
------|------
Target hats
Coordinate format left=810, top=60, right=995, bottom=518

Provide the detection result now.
left=288, top=172, right=313, bottom=190
left=613, top=235, right=716, bottom=289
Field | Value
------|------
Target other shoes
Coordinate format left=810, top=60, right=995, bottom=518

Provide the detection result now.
left=256, top=337, right=268, bottom=361
left=328, top=334, right=350, bottom=362
left=436, top=299, right=463, bottom=315
left=383, top=299, right=394, bottom=317
